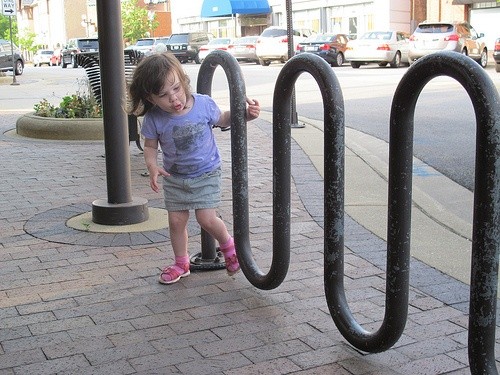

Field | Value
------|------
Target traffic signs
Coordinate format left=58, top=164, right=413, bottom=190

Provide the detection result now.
left=2, top=0, right=17, bottom=16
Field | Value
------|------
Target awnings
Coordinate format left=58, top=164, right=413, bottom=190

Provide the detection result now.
left=201, top=0, right=270, bottom=17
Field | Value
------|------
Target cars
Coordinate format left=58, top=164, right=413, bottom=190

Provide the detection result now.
left=228, top=36, right=259, bottom=65
left=0, top=38, right=26, bottom=76
left=198, top=37, right=232, bottom=64
left=492, top=37, right=500, bottom=72
left=343, top=31, right=411, bottom=68
left=295, top=33, right=350, bottom=67
left=32, top=49, right=53, bottom=67
left=49, top=47, right=63, bottom=66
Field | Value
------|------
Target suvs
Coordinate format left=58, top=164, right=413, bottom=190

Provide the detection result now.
left=407, top=18, right=488, bottom=68
left=134, top=37, right=170, bottom=52
left=255, top=26, right=308, bottom=66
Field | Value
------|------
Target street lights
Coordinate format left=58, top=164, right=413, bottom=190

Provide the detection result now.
left=81, top=13, right=95, bottom=37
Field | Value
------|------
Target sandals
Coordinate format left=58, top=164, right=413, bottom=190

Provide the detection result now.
left=159, top=263, right=190, bottom=284
left=221, top=245, right=241, bottom=277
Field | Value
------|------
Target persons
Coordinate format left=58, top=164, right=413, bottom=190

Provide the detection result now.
left=129, top=54, right=261, bottom=283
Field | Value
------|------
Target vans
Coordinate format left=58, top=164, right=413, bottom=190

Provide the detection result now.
left=165, top=32, right=216, bottom=65
left=59, top=37, right=100, bottom=69
